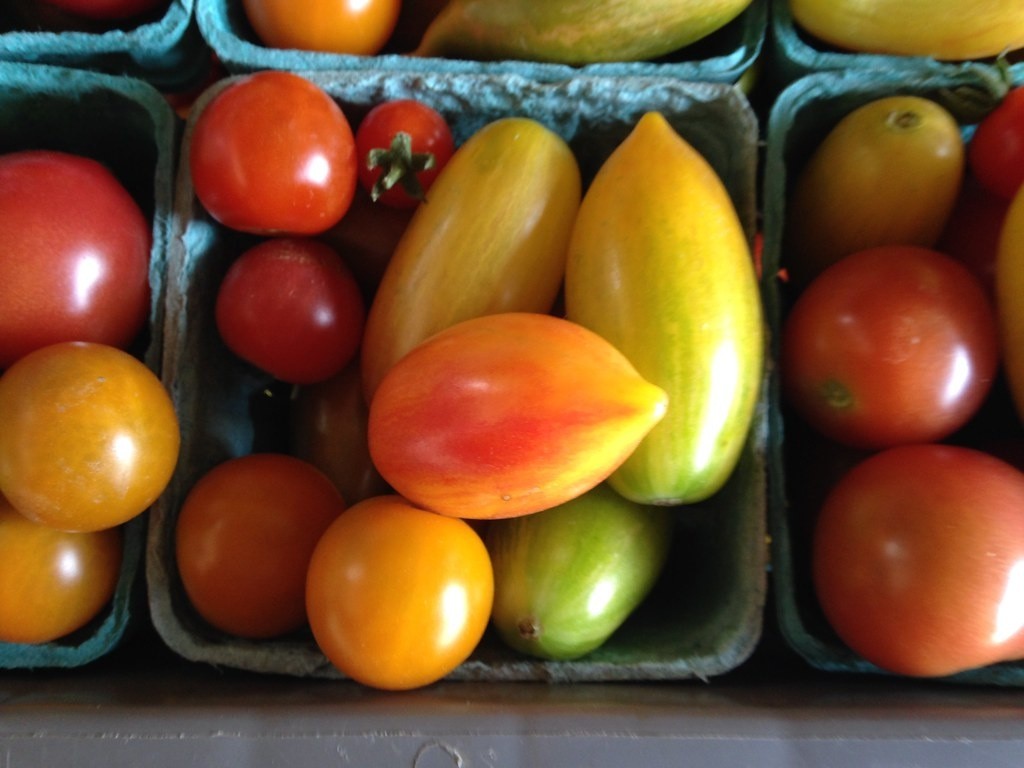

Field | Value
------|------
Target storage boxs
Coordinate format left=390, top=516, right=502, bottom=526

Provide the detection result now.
left=195, top=0, right=769, bottom=84
left=772, top=0, right=1024, bottom=86
left=763, top=61, right=1024, bottom=690
left=0, top=57, right=174, bottom=672
left=0, top=0, right=193, bottom=73
left=144, top=66, right=763, bottom=682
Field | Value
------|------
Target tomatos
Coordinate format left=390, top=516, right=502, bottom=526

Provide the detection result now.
left=1, top=0, right=1022, bottom=689
